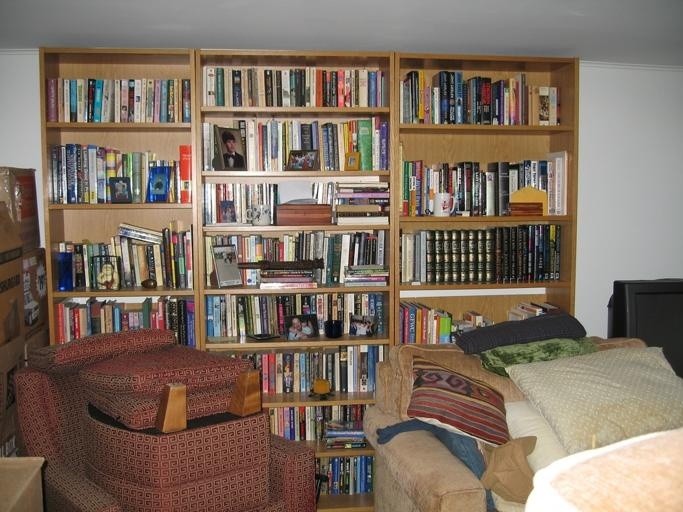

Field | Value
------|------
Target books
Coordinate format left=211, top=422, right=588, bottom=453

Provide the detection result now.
left=204, top=294, right=387, bottom=336
left=400, top=72, right=561, bottom=126
left=398, top=300, right=562, bottom=343
left=201, top=68, right=389, bottom=106
left=202, top=117, right=389, bottom=169
left=202, top=229, right=389, bottom=287
left=399, top=144, right=568, bottom=214
left=202, top=181, right=280, bottom=223
left=44, top=143, right=191, bottom=204
left=311, top=181, right=391, bottom=223
left=226, top=344, right=388, bottom=395
left=315, top=455, right=375, bottom=496
left=268, top=404, right=373, bottom=449
left=398, top=223, right=562, bottom=284
left=57, top=298, right=197, bottom=343
left=40, top=76, right=193, bottom=122
left=50, top=218, right=193, bottom=290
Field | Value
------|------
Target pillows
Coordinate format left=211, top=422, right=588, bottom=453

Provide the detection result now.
left=456, top=311, right=589, bottom=354
left=478, top=337, right=596, bottom=376
left=406, top=351, right=511, bottom=448
left=501, top=347, right=683, bottom=454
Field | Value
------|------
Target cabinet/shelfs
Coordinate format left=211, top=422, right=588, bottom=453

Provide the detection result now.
left=35, top=45, right=199, bottom=355
left=396, top=51, right=580, bottom=353
left=193, top=47, right=395, bottom=510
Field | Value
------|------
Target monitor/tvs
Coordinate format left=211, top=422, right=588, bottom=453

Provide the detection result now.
left=606, top=278, right=683, bottom=382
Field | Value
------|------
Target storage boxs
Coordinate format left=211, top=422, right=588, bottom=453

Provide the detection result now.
left=0, top=165, right=50, bottom=457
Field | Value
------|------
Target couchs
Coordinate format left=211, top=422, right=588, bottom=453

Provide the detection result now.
left=359, top=337, right=683, bottom=512
left=77, top=347, right=274, bottom=512
left=11, top=329, right=319, bottom=511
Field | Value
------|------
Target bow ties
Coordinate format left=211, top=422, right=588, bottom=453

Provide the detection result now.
left=226, top=154, right=235, bottom=159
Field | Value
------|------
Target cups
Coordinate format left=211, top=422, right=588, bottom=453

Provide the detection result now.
left=433, top=192, right=457, bottom=217
left=325, top=319, right=342, bottom=338
